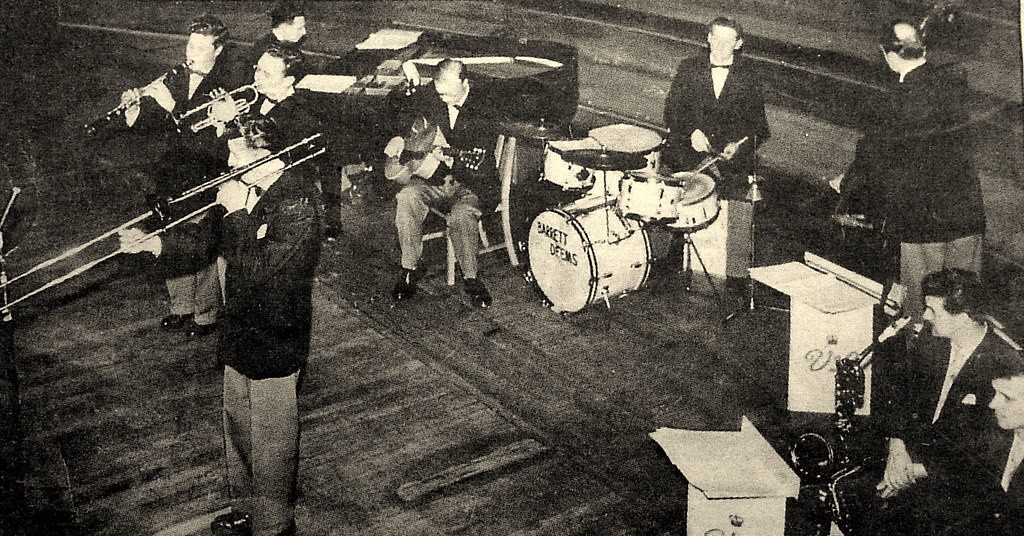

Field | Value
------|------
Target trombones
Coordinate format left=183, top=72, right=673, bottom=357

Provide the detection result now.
left=0, top=133, right=325, bottom=313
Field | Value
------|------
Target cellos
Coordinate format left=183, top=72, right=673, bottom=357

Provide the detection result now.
left=803, top=1, right=965, bottom=283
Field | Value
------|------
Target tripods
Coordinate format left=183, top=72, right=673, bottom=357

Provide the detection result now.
left=667, top=233, right=717, bottom=295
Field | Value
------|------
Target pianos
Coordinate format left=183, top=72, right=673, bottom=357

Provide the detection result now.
left=293, top=20, right=577, bottom=171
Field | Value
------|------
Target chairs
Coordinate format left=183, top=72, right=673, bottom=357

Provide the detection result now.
left=421, top=134, right=520, bottom=285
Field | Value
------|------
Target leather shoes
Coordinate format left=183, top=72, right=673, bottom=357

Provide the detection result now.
left=161, top=311, right=193, bottom=331
left=210, top=509, right=253, bottom=536
left=186, top=322, right=217, bottom=338
left=393, top=259, right=426, bottom=301
left=463, top=277, right=493, bottom=308
left=274, top=519, right=299, bottom=536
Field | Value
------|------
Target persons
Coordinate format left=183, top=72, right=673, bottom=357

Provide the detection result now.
left=209, top=45, right=323, bottom=185
left=829, top=22, right=986, bottom=349
left=118, top=112, right=320, bottom=535
left=988, top=349, right=1024, bottom=536
left=384, top=59, right=494, bottom=309
left=652, top=16, right=770, bottom=276
left=829, top=266, right=1008, bottom=536
left=248, top=1, right=317, bottom=113
left=121, top=15, right=246, bottom=338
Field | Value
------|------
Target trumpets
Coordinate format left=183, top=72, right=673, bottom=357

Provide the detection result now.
left=178, top=81, right=261, bottom=129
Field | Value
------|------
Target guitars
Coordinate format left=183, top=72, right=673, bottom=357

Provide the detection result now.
left=385, top=126, right=487, bottom=182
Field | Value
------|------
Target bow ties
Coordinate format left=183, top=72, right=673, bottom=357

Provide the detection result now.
left=710, top=63, right=731, bottom=69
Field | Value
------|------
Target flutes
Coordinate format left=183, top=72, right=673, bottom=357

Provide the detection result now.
left=77, top=61, right=192, bottom=133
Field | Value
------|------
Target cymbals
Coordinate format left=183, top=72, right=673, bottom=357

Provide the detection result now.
left=560, top=149, right=649, bottom=172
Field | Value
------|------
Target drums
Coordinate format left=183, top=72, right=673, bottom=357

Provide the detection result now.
left=541, top=136, right=600, bottom=192
left=657, top=170, right=721, bottom=235
left=526, top=196, right=653, bottom=320
left=587, top=122, right=665, bottom=174
left=617, top=170, right=683, bottom=224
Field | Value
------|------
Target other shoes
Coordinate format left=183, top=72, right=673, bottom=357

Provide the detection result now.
left=324, top=225, right=339, bottom=243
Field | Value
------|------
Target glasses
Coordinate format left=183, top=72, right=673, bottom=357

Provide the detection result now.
left=253, top=65, right=287, bottom=80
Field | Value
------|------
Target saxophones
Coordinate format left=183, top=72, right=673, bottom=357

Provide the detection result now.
left=791, top=314, right=913, bottom=536
